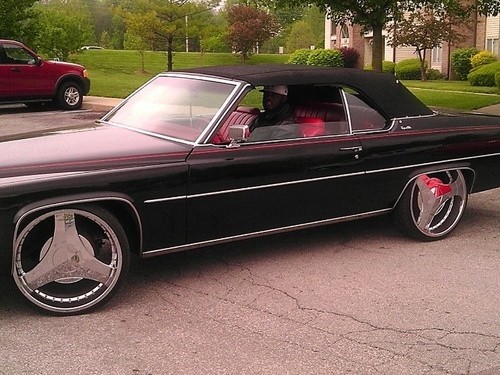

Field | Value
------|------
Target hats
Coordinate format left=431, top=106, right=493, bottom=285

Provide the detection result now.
left=258, top=85, right=289, bottom=96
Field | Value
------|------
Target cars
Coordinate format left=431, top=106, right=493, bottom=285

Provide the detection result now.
left=80, top=45, right=104, bottom=49
left=0, top=39, right=90, bottom=109
left=0, top=63, right=500, bottom=316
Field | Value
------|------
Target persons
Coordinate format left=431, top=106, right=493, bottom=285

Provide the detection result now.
left=250, top=85, right=297, bottom=141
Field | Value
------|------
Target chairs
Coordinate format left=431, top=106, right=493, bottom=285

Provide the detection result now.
left=293, top=105, right=374, bottom=138
left=214, top=105, right=261, bottom=144
